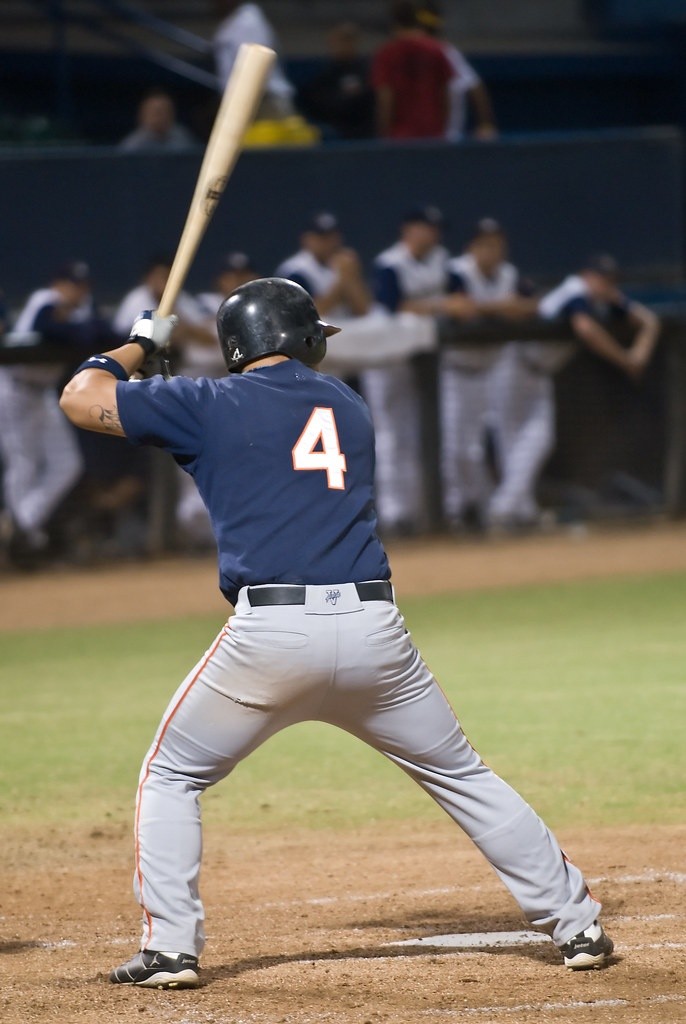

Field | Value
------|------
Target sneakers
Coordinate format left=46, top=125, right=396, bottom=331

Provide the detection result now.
left=555, top=920, right=614, bottom=968
left=108, top=949, right=202, bottom=992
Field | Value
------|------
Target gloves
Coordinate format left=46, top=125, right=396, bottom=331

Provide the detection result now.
left=125, top=309, right=179, bottom=359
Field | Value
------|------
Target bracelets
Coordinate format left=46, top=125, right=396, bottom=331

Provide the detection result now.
left=125, top=335, right=154, bottom=358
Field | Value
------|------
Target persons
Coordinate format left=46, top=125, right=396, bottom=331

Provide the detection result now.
left=0, top=0, right=657, bottom=567
left=57, top=275, right=615, bottom=987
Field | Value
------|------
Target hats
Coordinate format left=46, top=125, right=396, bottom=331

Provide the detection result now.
left=54, top=202, right=632, bottom=289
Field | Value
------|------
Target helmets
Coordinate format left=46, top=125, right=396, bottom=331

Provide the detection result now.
left=216, top=277, right=341, bottom=374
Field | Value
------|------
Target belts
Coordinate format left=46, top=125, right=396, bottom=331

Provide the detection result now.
left=247, top=582, right=395, bottom=608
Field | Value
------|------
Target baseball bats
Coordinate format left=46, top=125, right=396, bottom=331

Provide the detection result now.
left=139, top=40, right=278, bottom=369
